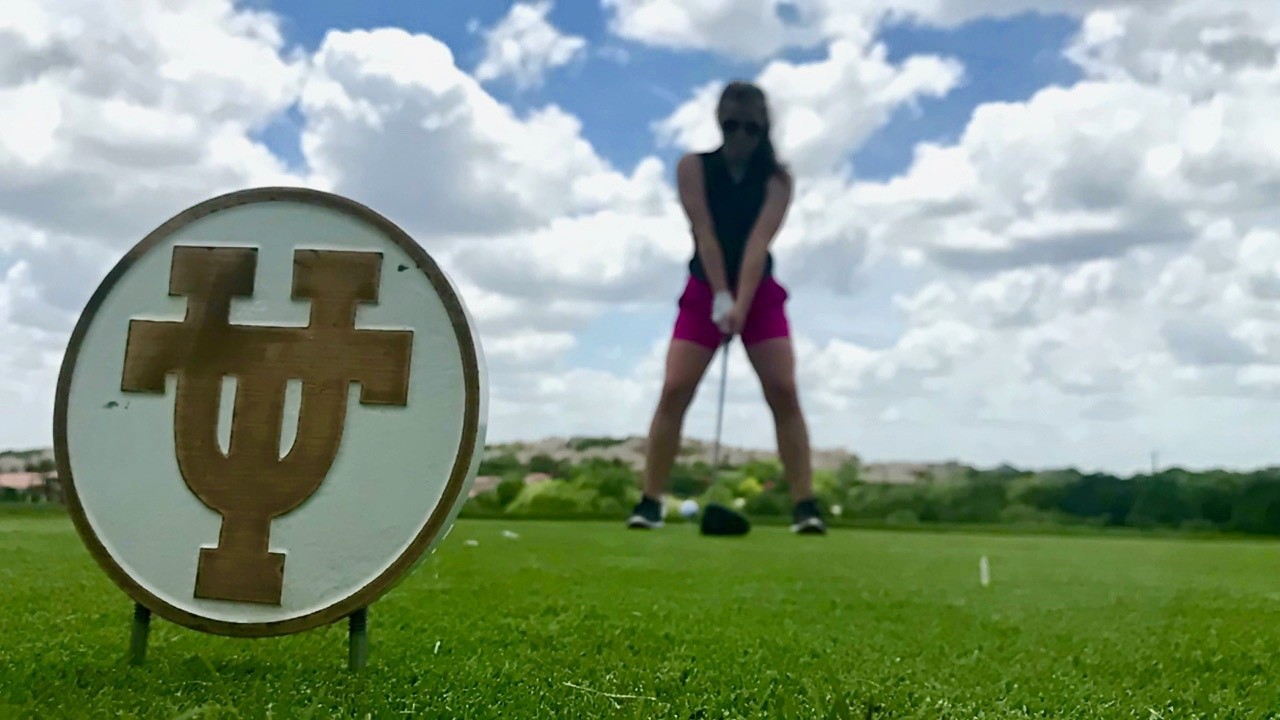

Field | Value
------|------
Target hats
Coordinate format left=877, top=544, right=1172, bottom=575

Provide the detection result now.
left=716, top=84, right=767, bottom=128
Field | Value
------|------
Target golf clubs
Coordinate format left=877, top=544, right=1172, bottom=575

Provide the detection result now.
left=701, top=333, right=751, bottom=538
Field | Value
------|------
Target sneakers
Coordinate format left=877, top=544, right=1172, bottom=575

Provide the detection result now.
left=792, top=497, right=828, bottom=534
left=626, top=495, right=664, bottom=527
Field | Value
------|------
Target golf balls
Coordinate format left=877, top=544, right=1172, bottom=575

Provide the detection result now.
left=679, top=500, right=699, bottom=519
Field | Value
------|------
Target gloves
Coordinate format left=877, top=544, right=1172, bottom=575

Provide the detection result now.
left=712, top=288, right=736, bottom=334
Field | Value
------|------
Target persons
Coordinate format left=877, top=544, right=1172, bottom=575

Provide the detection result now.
left=627, top=79, right=826, bottom=536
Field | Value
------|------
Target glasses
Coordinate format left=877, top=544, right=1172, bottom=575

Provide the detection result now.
left=721, top=120, right=759, bottom=135
